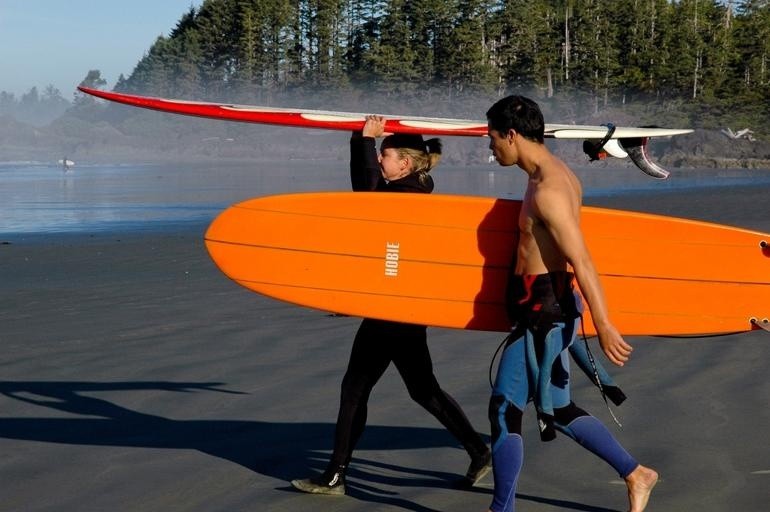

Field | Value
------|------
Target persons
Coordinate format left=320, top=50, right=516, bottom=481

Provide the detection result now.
left=287, top=113, right=494, bottom=497
left=484, top=92, right=660, bottom=512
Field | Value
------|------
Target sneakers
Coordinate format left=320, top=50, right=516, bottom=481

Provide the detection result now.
left=291, top=478, right=346, bottom=495
left=466, top=448, right=492, bottom=486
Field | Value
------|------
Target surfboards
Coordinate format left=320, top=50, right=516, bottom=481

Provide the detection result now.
left=204, top=191, right=770, bottom=337
left=77, top=86, right=693, bottom=180
left=59, top=160, right=74, bottom=166
